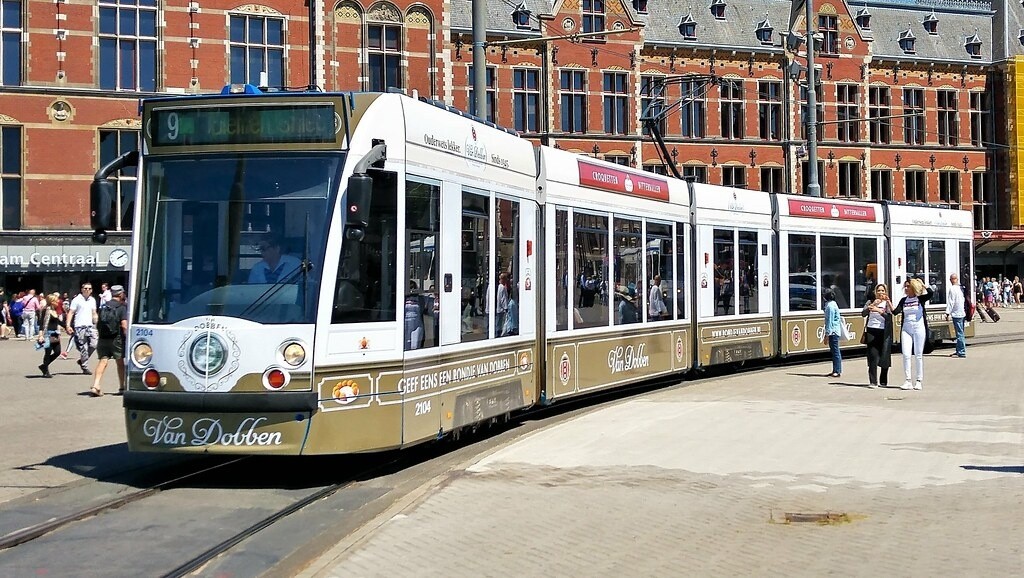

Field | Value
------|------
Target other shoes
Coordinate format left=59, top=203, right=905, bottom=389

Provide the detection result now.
left=867, top=383, right=878, bottom=388
left=77, top=359, right=92, bottom=375
left=60, top=352, right=67, bottom=359
left=39, top=364, right=52, bottom=378
left=878, top=383, right=887, bottom=387
left=828, top=372, right=842, bottom=378
left=950, top=353, right=967, bottom=358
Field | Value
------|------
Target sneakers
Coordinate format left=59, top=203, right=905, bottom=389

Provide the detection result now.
left=901, top=380, right=913, bottom=390
left=913, top=381, right=922, bottom=390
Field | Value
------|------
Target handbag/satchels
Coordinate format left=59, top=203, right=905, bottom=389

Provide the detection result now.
left=839, top=322, right=850, bottom=342
left=50, top=333, right=61, bottom=343
left=861, top=332, right=867, bottom=344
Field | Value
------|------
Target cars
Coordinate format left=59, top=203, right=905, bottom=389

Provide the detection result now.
left=789, top=272, right=846, bottom=308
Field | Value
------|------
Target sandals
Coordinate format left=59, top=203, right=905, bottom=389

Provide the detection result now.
left=90, top=387, right=125, bottom=396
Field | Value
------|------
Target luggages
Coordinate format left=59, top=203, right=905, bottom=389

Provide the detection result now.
left=981, top=302, right=1000, bottom=322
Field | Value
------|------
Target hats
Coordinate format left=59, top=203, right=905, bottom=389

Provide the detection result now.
left=111, top=285, right=125, bottom=296
left=910, top=279, right=923, bottom=294
left=615, top=286, right=632, bottom=301
left=40, top=293, right=44, bottom=297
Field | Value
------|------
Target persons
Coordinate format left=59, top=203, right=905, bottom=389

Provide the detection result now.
left=404, top=272, right=521, bottom=354
left=976, top=275, right=1023, bottom=323
left=557, top=269, right=667, bottom=329
left=861, top=283, right=894, bottom=388
left=249, top=232, right=305, bottom=288
left=719, top=269, right=752, bottom=315
left=945, top=273, right=968, bottom=358
left=822, top=287, right=843, bottom=378
left=865, top=272, right=876, bottom=303
left=886, top=278, right=933, bottom=391
left=0, top=282, right=127, bottom=399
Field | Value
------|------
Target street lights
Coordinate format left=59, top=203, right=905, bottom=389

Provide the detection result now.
left=787, top=0, right=826, bottom=196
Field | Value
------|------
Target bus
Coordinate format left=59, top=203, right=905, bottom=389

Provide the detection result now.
left=90, top=75, right=976, bottom=457
left=622, top=238, right=684, bottom=314
left=408, top=235, right=479, bottom=315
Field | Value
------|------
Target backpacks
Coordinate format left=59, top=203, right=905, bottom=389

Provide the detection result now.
left=964, top=295, right=975, bottom=322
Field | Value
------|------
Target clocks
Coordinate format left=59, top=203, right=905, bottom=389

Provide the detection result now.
left=109, top=248, right=129, bottom=268
